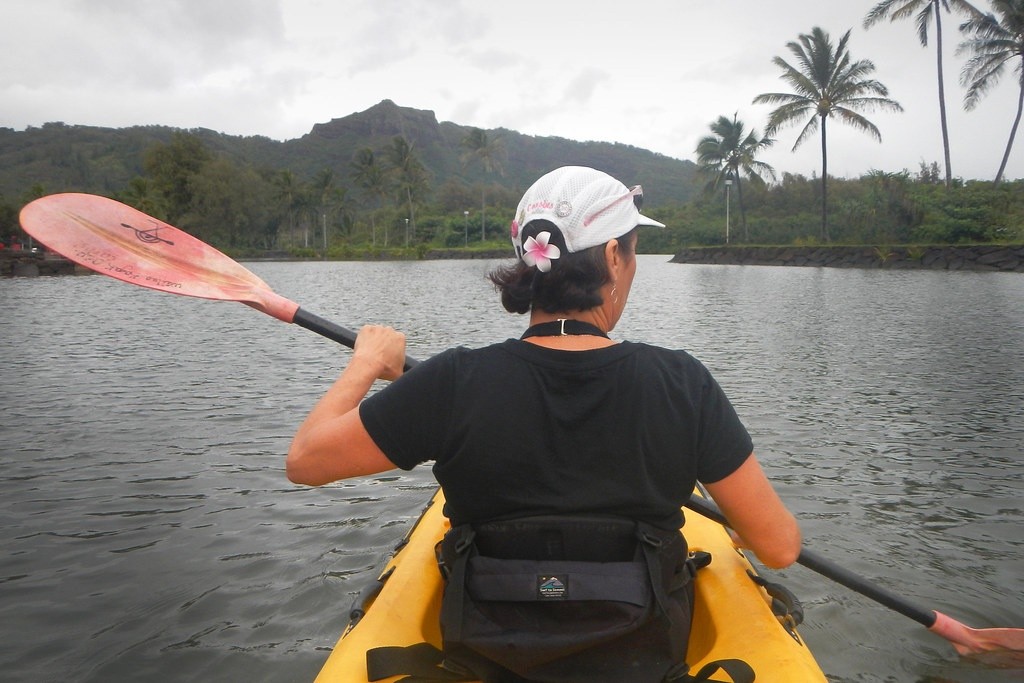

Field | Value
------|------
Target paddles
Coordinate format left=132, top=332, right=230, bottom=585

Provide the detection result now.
left=19, top=193, right=1024, bottom=656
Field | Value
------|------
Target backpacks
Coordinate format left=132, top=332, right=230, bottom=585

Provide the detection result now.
left=368, top=512, right=753, bottom=682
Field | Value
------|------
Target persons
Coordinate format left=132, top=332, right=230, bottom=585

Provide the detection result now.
left=284, top=164, right=805, bottom=683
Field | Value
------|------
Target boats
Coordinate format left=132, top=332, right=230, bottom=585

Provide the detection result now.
left=311, top=479, right=831, bottom=683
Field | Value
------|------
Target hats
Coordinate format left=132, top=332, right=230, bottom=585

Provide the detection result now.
left=510, top=165, right=667, bottom=262
